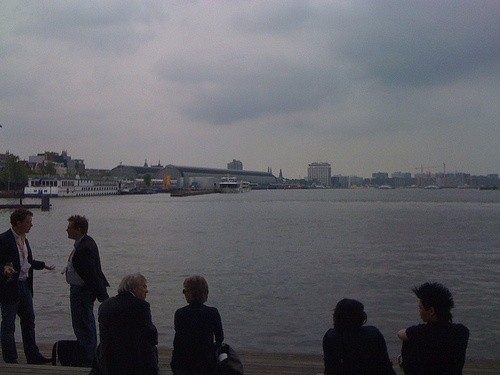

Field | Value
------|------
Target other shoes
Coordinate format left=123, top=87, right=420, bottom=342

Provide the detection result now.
left=4, top=359, right=17, bottom=363
left=27, top=357, right=53, bottom=365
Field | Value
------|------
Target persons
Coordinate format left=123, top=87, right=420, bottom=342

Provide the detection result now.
left=397, top=282, right=470, bottom=375
left=322, top=298, right=397, bottom=375
left=66, top=215, right=111, bottom=346
left=98, top=273, right=159, bottom=375
left=169, top=275, right=224, bottom=375
left=0, top=209, right=56, bottom=365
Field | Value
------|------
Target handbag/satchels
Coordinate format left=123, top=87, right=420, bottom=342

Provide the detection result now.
left=214, top=343, right=243, bottom=375
left=51, top=339, right=91, bottom=368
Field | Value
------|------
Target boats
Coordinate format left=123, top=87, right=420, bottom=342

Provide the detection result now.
left=218, top=176, right=241, bottom=194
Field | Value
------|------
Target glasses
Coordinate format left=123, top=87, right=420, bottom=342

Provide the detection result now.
left=183, top=288, right=188, bottom=294
